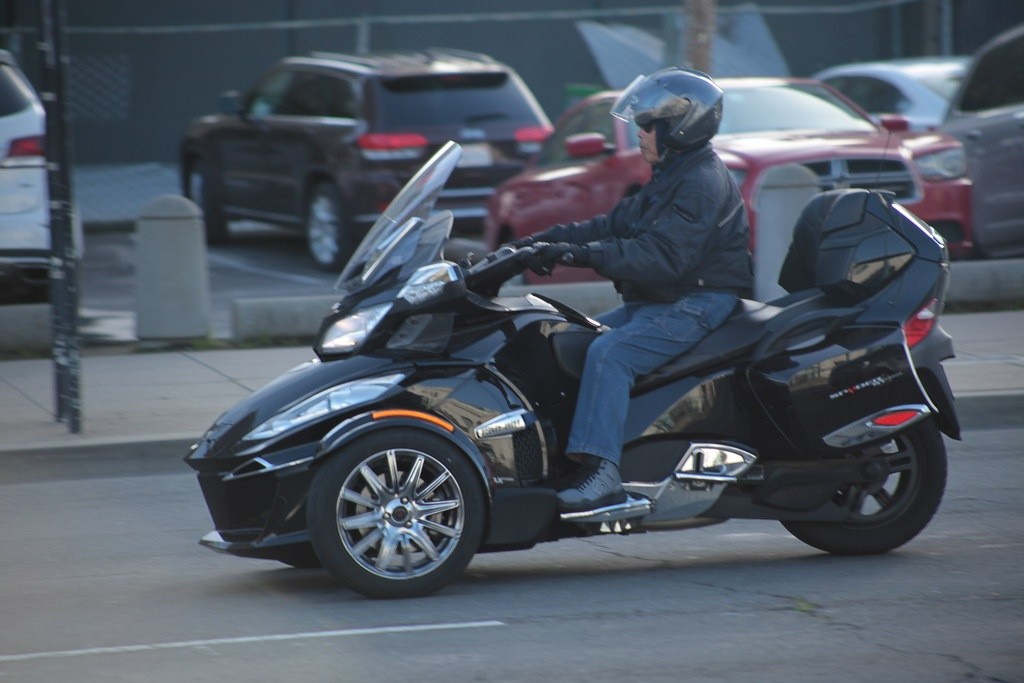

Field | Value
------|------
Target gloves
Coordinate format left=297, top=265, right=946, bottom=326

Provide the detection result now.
left=499, top=236, right=534, bottom=249
left=528, top=242, right=587, bottom=275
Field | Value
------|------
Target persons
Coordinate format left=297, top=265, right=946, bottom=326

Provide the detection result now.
left=499, top=66, right=754, bottom=510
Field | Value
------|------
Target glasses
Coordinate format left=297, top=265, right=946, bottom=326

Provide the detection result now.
left=634, top=111, right=661, bottom=127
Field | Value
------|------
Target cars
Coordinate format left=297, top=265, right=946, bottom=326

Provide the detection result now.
left=485, top=77, right=975, bottom=288
left=0, top=49, right=84, bottom=303
left=816, top=59, right=968, bottom=136
left=937, top=24, right=1023, bottom=254
left=181, top=48, right=554, bottom=272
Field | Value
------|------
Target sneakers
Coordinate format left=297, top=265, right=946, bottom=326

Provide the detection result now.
left=557, top=459, right=627, bottom=512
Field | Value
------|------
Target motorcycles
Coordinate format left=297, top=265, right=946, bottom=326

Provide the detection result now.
left=182, top=141, right=961, bottom=600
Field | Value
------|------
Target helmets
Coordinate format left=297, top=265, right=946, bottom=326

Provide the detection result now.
left=629, top=66, right=724, bottom=154
